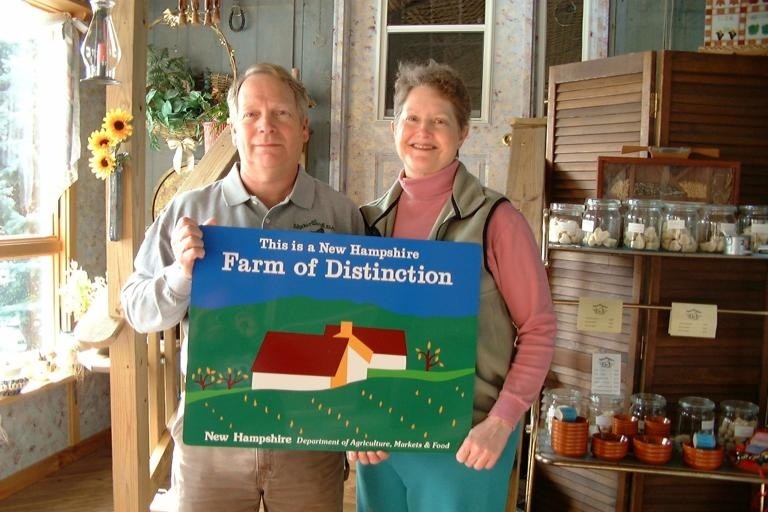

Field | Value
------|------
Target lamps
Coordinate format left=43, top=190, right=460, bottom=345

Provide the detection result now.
left=76, top=0, right=124, bottom=91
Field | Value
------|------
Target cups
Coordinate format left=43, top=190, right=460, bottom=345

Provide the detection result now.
left=549, top=418, right=588, bottom=459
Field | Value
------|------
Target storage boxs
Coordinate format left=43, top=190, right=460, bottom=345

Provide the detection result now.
left=593, top=154, right=741, bottom=211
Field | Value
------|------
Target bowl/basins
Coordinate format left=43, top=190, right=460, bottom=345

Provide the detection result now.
left=590, top=434, right=628, bottom=461
left=634, top=437, right=671, bottom=465
left=682, top=443, right=724, bottom=472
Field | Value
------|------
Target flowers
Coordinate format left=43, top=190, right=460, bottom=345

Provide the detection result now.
left=85, top=104, right=138, bottom=181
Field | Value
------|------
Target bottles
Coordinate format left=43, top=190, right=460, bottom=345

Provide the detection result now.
left=546, top=199, right=767, bottom=255
left=543, top=389, right=760, bottom=448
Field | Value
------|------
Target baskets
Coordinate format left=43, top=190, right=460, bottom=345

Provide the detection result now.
left=139, top=8, right=237, bottom=145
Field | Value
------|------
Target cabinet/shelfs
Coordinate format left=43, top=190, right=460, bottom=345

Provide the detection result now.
left=521, top=197, right=768, bottom=510
left=498, top=114, right=547, bottom=264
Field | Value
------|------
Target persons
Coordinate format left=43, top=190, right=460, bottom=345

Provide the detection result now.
left=120, top=63, right=389, bottom=510
left=356, top=59, right=558, bottom=510
